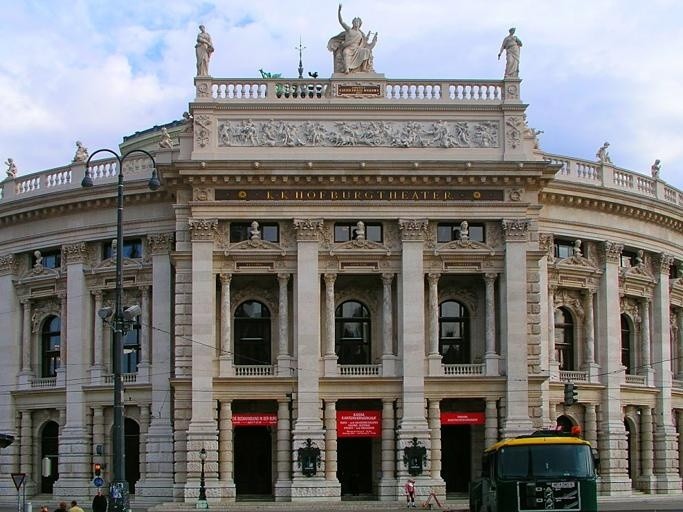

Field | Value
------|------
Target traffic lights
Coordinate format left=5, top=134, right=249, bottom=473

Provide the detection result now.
left=564, top=382, right=579, bottom=406
left=95, top=462, right=101, bottom=477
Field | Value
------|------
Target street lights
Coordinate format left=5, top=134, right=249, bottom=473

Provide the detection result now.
left=193, top=447, right=209, bottom=508
left=80, top=147, right=160, bottom=512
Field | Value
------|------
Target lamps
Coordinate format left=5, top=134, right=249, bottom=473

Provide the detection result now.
left=294, top=437, right=324, bottom=477
left=403, top=437, right=429, bottom=477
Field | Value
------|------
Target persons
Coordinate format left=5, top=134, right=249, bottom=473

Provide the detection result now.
left=498, top=28, right=523, bottom=81
left=573, top=239, right=582, bottom=257
left=158, top=127, right=176, bottom=149
left=218, top=119, right=495, bottom=148
left=596, top=140, right=613, bottom=162
left=39, top=503, right=48, bottom=512
left=34, top=251, right=43, bottom=264
left=250, top=221, right=261, bottom=239
left=635, top=250, right=644, bottom=263
left=459, top=221, right=468, bottom=238
left=355, top=221, right=365, bottom=240
left=651, top=159, right=662, bottom=179
left=71, top=141, right=89, bottom=162
left=404, top=477, right=417, bottom=509
left=53, top=501, right=68, bottom=511
left=67, top=500, right=84, bottom=511
left=3, top=158, right=18, bottom=179
left=193, top=24, right=215, bottom=77
left=91, top=488, right=108, bottom=511
left=336, top=4, right=376, bottom=75
left=112, top=239, right=118, bottom=256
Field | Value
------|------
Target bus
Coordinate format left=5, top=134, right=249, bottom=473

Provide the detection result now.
left=479, top=426, right=602, bottom=512
